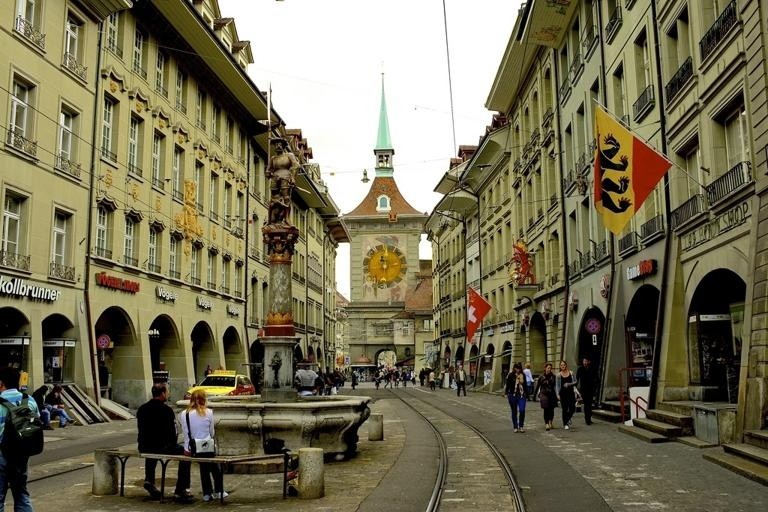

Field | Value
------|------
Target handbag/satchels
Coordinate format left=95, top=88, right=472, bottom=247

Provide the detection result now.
left=190, top=438, right=215, bottom=458
left=264, top=439, right=284, bottom=453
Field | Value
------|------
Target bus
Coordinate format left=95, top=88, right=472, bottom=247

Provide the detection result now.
left=351, top=365, right=377, bottom=379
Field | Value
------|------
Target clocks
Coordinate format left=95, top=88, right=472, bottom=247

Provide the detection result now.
left=364, top=243, right=406, bottom=288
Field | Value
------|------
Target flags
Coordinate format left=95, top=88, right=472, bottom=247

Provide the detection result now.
left=593, top=104, right=672, bottom=237
left=465, top=287, right=492, bottom=344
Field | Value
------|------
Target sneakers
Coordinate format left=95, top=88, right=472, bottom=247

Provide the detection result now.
left=174, top=489, right=193, bottom=497
left=203, top=494, right=213, bottom=501
left=143, top=481, right=161, bottom=495
left=215, top=491, right=229, bottom=499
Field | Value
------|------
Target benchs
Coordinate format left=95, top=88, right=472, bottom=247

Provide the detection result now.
left=109, top=443, right=291, bottom=502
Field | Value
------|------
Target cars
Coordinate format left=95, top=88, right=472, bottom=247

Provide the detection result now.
left=183, top=368, right=257, bottom=401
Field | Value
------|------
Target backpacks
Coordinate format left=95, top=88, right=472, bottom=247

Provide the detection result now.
left=0, top=393, right=43, bottom=458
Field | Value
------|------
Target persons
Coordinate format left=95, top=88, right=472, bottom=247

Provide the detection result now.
left=504, top=356, right=599, bottom=433
left=181, top=390, right=229, bottom=501
left=135, top=384, right=193, bottom=499
left=454, top=365, right=467, bottom=397
left=266, top=136, right=298, bottom=205
left=0, top=366, right=41, bottom=512
left=311, top=365, right=446, bottom=396
left=44, top=384, right=74, bottom=429
left=18, top=369, right=28, bottom=392
left=99, top=360, right=108, bottom=398
left=31, top=385, right=54, bottom=430
left=204, top=364, right=213, bottom=377
